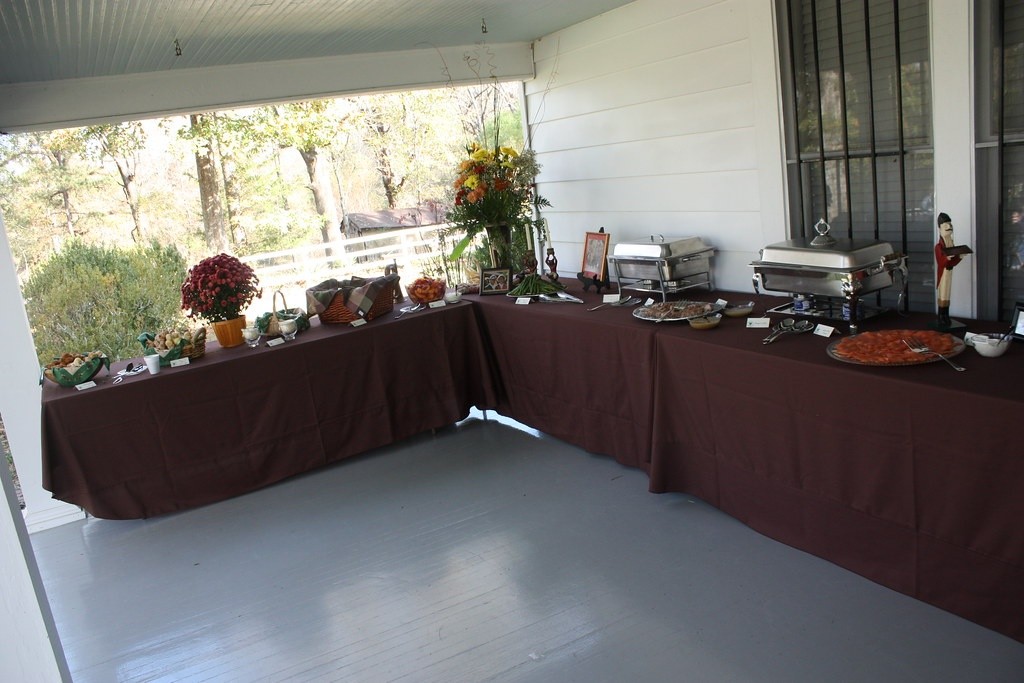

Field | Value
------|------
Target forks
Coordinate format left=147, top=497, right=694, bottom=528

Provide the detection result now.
left=902, top=337, right=966, bottom=371
left=655, top=299, right=689, bottom=323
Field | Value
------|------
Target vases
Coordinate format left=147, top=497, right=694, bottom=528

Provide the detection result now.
left=482, top=221, right=513, bottom=267
left=210, top=315, right=247, bottom=347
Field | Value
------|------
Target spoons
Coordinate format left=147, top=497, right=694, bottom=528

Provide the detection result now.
left=763, top=320, right=808, bottom=344
left=763, top=318, right=795, bottom=341
left=394, top=303, right=421, bottom=318
left=586, top=295, right=631, bottom=311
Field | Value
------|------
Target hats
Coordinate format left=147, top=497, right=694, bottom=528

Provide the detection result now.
left=489, top=275, right=497, bottom=279
left=497, top=274, right=506, bottom=282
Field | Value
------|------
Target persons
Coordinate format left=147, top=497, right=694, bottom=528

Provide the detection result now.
left=485, top=275, right=507, bottom=290
left=935, top=212, right=970, bottom=325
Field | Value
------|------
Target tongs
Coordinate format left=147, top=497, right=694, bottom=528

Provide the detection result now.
left=536, top=292, right=583, bottom=304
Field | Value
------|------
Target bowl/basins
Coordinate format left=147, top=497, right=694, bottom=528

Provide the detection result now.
left=446, top=293, right=462, bottom=303
left=969, top=332, right=1013, bottom=357
left=687, top=312, right=722, bottom=328
left=722, top=300, right=755, bottom=316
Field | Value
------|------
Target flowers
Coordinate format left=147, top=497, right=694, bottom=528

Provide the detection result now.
left=180, top=253, right=263, bottom=327
left=439, top=142, right=554, bottom=261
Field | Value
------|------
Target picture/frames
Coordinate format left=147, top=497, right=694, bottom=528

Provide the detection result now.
left=581, top=231, right=610, bottom=282
left=478, top=268, right=512, bottom=296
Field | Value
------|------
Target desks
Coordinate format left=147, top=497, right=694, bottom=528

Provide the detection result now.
left=40, top=289, right=498, bottom=521
left=460, top=276, right=1024, bottom=644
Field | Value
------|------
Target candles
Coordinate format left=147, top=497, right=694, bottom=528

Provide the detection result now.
left=543, top=219, right=551, bottom=249
left=524, top=223, right=533, bottom=250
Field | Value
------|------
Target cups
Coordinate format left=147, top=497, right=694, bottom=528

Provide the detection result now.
left=279, top=324, right=297, bottom=340
left=143, top=354, right=160, bottom=374
left=243, top=330, right=260, bottom=347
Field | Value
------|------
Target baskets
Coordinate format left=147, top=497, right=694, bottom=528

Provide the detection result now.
left=305, top=273, right=400, bottom=322
left=42, top=349, right=104, bottom=386
left=254, top=290, right=310, bottom=336
left=137, top=327, right=207, bottom=360
left=405, top=277, right=447, bottom=304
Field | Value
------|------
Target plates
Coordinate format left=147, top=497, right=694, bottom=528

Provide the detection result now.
left=400, top=305, right=426, bottom=313
left=507, top=283, right=567, bottom=297
left=610, top=298, right=642, bottom=307
left=826, top=328, right=965, bottom=365
left=118, top=366, right=147, bottom=376
left=632, top=300, right=723, bottom=321
left=773, top=321, right=814, bottom=333
